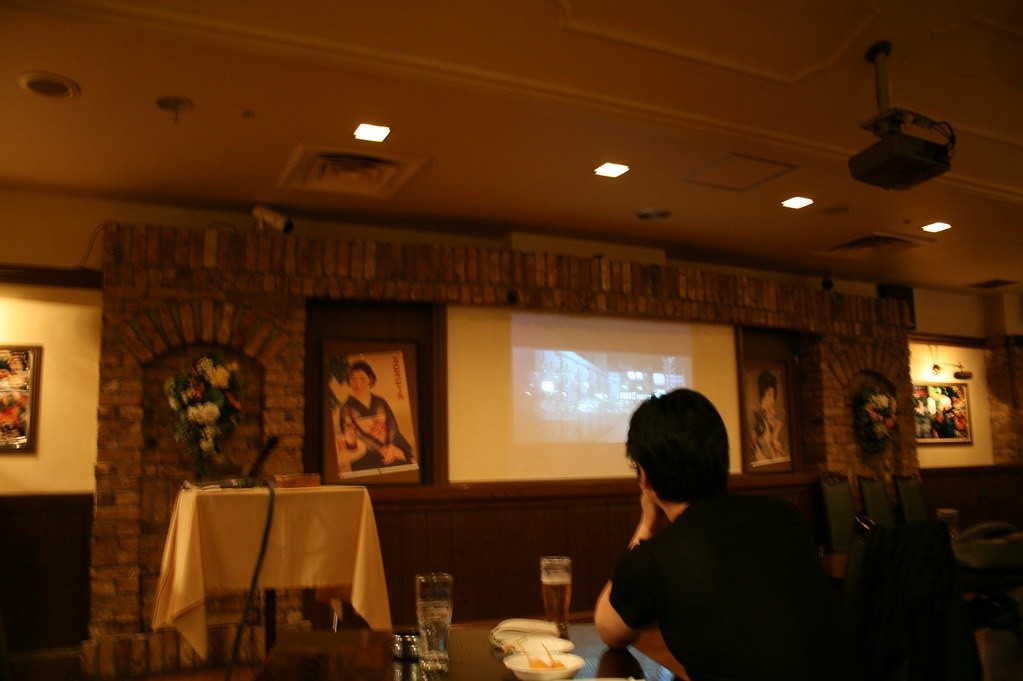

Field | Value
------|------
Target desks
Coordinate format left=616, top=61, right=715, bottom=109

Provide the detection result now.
left=420, top=617, right=682, bottom=681
left=177, top=485, right=369, bottom=658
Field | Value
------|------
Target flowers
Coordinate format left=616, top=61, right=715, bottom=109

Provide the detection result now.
left=866, top=390, right=898, bottom=440
left=162, top=355, right=248, bottom=461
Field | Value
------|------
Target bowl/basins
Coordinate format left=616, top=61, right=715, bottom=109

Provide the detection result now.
left=502, top=653, right=586, bottom=681
left=501, top=636, right=575, bottom=653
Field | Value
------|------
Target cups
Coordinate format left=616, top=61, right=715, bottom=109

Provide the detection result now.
left=415, top=573, right=454, bottom=675
left=541, top=556, right=573, bottom=641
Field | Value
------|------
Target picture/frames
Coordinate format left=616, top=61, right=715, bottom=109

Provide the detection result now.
left=742, top=358, right=795, bottom=475
left=0, top=345, right=43, bottom=452
left=912, top=381, right=974, bottom=444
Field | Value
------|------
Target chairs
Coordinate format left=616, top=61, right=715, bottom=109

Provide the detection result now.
left=857, top=475, right=896, bottom=528
left=842, top=519, right=951, bottom=681
left=818, top=475, right=859, bottom=554
left=893, top=473, right=931, bottom=525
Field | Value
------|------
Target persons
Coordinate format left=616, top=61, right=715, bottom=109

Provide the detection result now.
left=594, top=389, right=863, bottom=681
